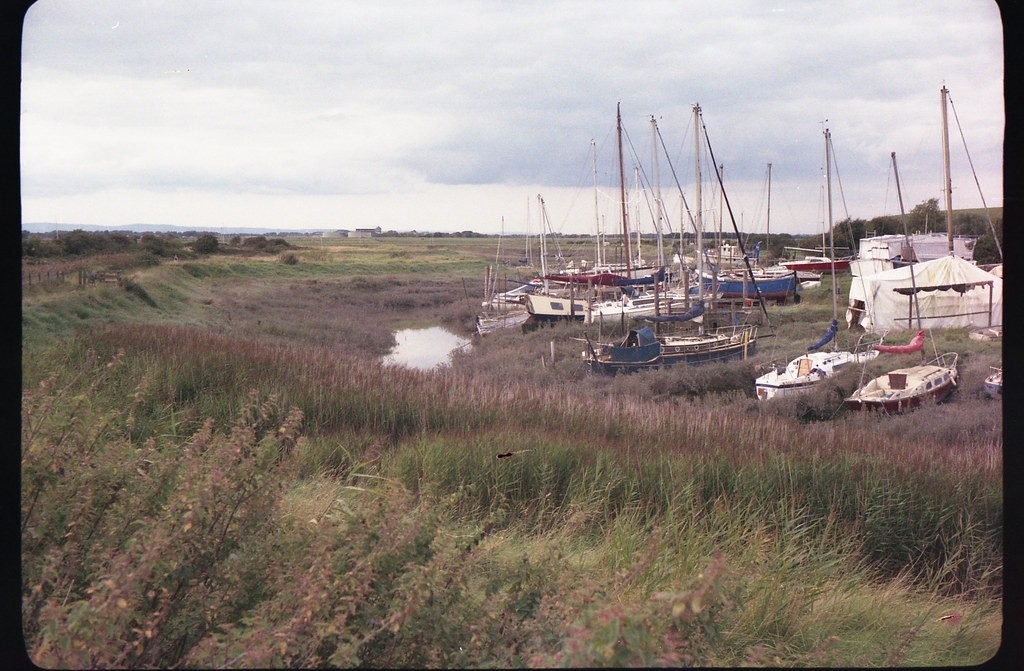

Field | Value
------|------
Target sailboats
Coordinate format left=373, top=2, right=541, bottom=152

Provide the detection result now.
left=470, top=84, right=927, bottom=372
left=755, top=129, right=884, bottom=402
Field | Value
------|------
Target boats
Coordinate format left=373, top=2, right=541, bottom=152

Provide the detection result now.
left=984, top=368, right=1001, bottom=401
left=842, top=350, right=960, bottom=414
